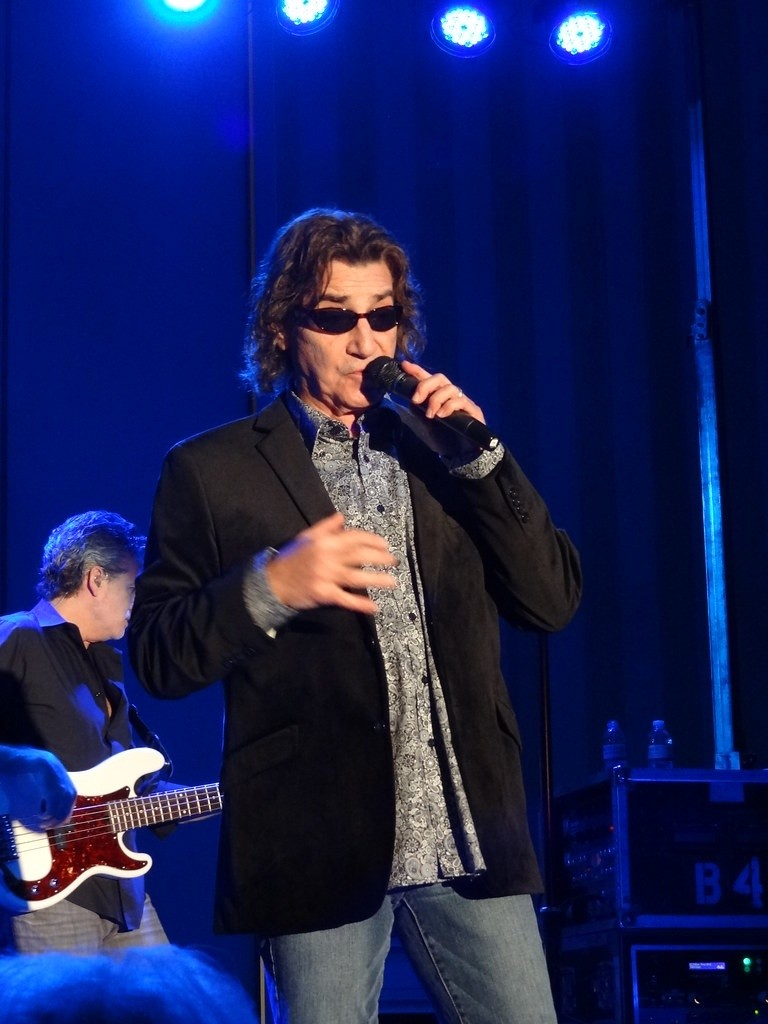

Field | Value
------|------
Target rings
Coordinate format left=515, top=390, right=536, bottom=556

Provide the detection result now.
left=457, top=386, right=463, bottom=397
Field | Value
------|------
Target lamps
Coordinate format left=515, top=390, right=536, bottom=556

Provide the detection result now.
left=429, top=3, right=499, bottom=59
left=546, top=6, right=615, bottom=66
left=276, top=0, right=340, bottom=38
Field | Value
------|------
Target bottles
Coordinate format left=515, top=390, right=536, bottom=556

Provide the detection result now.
left=603, top=720, right=628, bottom=777
left=647, top=719, right=674, bottom=769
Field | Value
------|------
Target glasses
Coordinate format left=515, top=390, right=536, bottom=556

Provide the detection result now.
left=306, top=305, right=404, bottom=335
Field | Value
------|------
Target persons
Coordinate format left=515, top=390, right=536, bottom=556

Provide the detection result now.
left=125, top=210, right=584, bottom=1024
left=1, top=511, right=172, bottom=958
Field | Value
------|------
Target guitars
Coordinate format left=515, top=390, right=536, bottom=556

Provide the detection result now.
left=0, top=745, right=229, bottom=916
